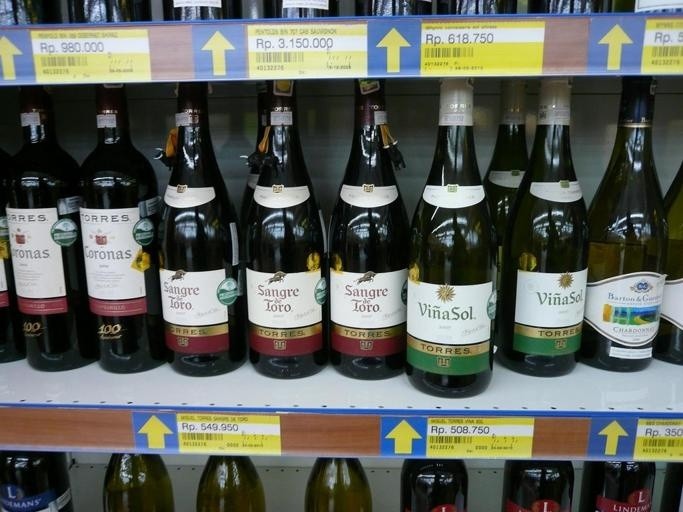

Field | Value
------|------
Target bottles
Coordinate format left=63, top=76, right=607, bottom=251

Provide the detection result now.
left=0, top=0, right=683, bottom=511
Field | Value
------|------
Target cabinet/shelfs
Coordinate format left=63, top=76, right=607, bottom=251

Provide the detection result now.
left=0, top=0, right=683, bottom=512
